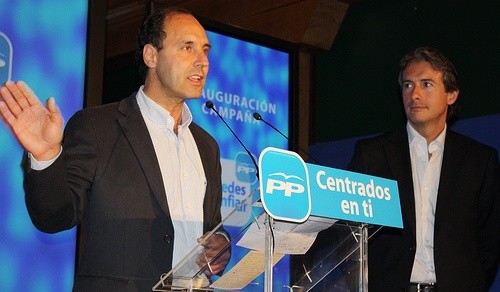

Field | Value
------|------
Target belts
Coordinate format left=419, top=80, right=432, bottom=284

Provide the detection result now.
left=409, top=282, right=436, bottom=292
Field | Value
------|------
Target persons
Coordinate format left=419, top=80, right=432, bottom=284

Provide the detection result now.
left=345, top=48, right=500, bottom=292
left=0, top=8, right=232, bottom=292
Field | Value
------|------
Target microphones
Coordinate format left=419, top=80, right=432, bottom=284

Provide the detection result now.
left=206, top=100, right=259, bottom=179
left=252, top=112, right=324, bottom=165
